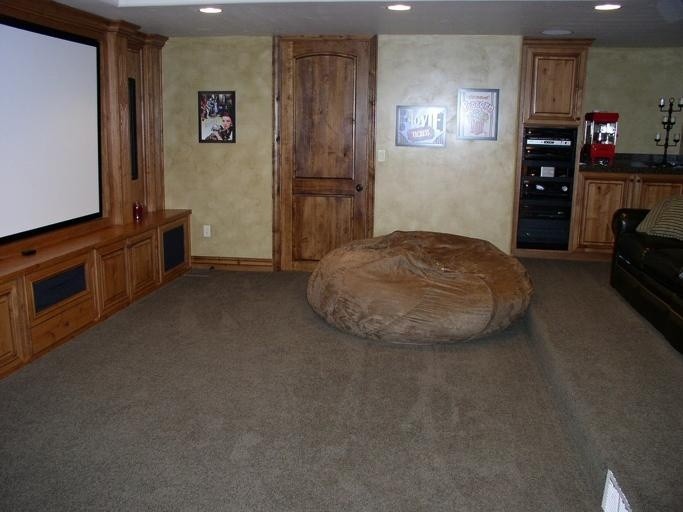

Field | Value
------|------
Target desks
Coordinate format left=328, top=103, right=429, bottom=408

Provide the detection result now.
left=572, top=153, right=683, bottom=263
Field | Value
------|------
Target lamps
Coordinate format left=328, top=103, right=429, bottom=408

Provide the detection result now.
left=653, top=96, right=683, bottom=168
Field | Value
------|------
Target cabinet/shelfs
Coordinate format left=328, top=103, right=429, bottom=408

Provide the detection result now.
left=1, top=209, right=191, bottom=379
left=517, top=36, right=593, bottom=128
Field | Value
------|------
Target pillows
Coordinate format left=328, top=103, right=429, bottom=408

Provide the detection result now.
left=635, top=195, right=683, bottom=241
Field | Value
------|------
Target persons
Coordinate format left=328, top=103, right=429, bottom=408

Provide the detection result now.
left=213, top=112, right=233, bottom=140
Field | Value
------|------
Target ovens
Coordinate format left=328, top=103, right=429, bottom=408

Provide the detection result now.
left=518, top=125, right=576, bottom=251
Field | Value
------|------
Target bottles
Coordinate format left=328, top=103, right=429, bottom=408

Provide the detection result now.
left=134, top=198, right=141, bottom=220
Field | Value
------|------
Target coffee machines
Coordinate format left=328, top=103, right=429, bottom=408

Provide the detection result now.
left=580, top=110, right=620, bottom=168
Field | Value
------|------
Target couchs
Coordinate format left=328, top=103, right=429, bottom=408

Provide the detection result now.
left=610, top=206, right=682, bottom=354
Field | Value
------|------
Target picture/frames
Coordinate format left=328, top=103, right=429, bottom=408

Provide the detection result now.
left=394, top=104, right=449, bottom=150
left=454, top=87, right=499, bottom=142
left=196, top=89, right=237, bottom=145
